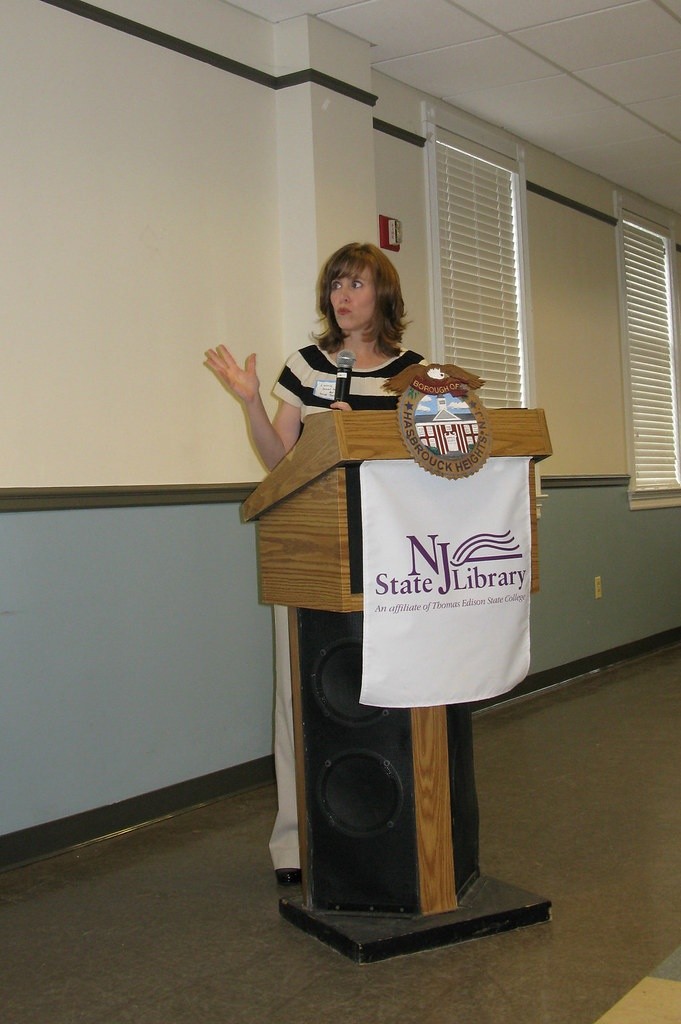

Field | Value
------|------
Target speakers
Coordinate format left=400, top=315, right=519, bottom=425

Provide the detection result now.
left=296, top=606, right=480, bottom=915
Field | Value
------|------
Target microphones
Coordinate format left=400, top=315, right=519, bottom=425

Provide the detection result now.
left=333, top=349, right=355, bottom=411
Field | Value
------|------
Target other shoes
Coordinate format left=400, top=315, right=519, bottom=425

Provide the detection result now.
left=275, top=866, right=300, bottom=885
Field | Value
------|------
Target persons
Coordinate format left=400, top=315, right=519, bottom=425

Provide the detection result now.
left=207, top=243, right=430, bottom=886
